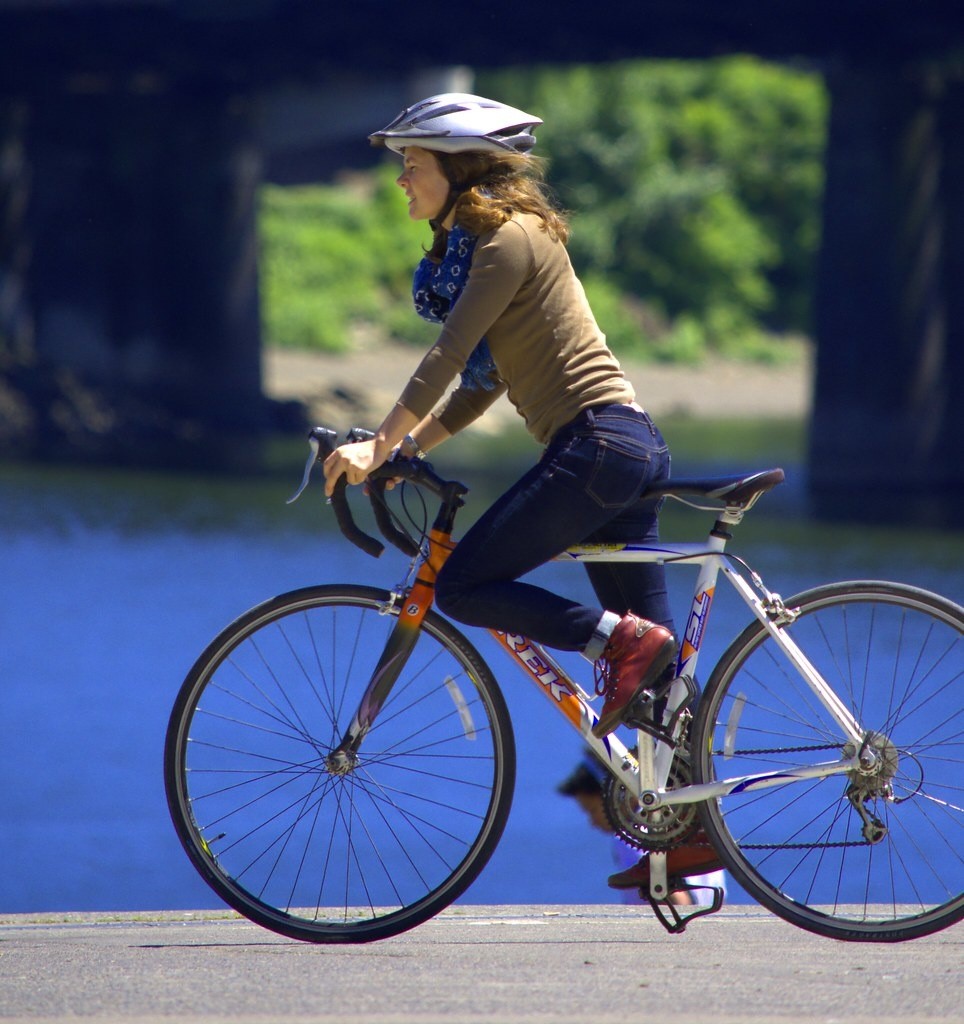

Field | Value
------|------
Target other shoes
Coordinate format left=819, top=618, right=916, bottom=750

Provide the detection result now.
left=592, top=608, right=679, bottom=739
left=607, top=829, right=730, bottom=888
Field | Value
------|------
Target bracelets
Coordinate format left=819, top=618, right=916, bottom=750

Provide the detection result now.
left=404, top=435, right=427, bottom=459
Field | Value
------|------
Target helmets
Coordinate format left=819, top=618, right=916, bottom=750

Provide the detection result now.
left=367, top=92, right=543, bottom=162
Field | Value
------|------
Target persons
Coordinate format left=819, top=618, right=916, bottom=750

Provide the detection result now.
left=554, top=746, right=727, bottom=905
left=323, top=91, right=725, bottom=889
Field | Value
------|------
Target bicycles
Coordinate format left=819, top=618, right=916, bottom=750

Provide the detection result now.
left=163, top=426, right=963, bottom=946
